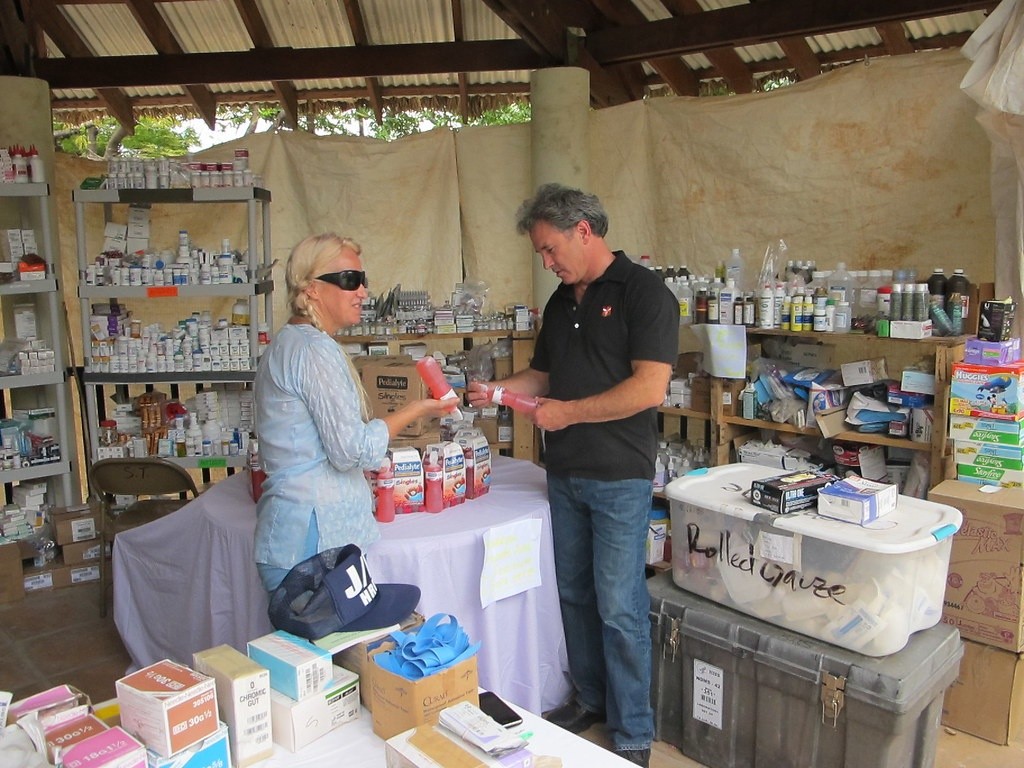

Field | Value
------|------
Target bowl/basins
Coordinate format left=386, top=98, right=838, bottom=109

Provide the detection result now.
left=650, top=504, right=667, bottom=519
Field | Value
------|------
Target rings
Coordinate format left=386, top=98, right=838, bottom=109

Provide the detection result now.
left=468, top=403, right=474, bottom=408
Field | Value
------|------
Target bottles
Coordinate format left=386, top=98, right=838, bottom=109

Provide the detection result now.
left=477, top=383, right=538, bottom=416
left=425, top=451, right=443, bottom=512
left=334, top=284, right=514, bottom=336
left=375, top=458, right=396, bottom=523
left=651, top=439, right=710, bottom=493
left=0, top=142, right=259, bottom=458
left=664, top=530, right=672, bottom=561
left=416, top=355, right=464, bottom=422
left=634, top=248, right=970, bottom=339
left=723, top=378, right=759, bottom=420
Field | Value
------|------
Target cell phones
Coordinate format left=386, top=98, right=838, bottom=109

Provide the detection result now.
left=479, top=691, right=523, bottom=728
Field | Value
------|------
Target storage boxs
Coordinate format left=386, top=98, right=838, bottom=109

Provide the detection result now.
left=841, top=356, right=890, bottom=386
left=473, top=418, right=499, bottom=444
left=385, top=448, right=425, bottom=514
left=671, top=375, right=757, bottom=422
left=962, top=281, right=996, bottom=333
left=352, top=354, right=440, bottom=459
left=454, top=428, right=493, bottom=498
left=950, top=302, right=1024, bottom=486
left=901, top=369, right=936, bottom=396
left=9, top=613, right=523, bottom=768
left=646, top=463, right=1024, bottom=768
left=426, top=440, right=467, bottom=506
left=0, top=499, right=116, bottom=604
left=496, top=356, right=514, bottom=378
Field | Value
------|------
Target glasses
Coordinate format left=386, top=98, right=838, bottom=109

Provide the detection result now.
left=304, top=269, right=367, bottom=292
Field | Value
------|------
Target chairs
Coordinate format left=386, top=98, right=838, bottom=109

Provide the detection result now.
left=89, top=458, right=200, bottom=616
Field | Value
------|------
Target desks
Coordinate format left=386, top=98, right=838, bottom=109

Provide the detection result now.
left=115, top=454, right=570, bottom=721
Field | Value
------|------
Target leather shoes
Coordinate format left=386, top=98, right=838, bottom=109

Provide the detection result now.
left=614, top=746, right=651, bottom=768
left=547, top=694, right=608, bottom=734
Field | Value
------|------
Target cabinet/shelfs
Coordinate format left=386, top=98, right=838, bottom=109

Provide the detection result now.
left=660, top=320, right=973, bottom=486
left=72, top=189, right=274, bottom=469
left=0, top=183, right=71, bottom=510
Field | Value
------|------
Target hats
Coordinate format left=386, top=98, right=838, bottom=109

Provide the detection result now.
left=266, top=543, right=420, bottom=641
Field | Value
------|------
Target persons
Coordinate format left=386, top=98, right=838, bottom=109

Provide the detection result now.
left=254, top=231, right=460, bottom=630
left=466, top=183, right=679, bottom=768
